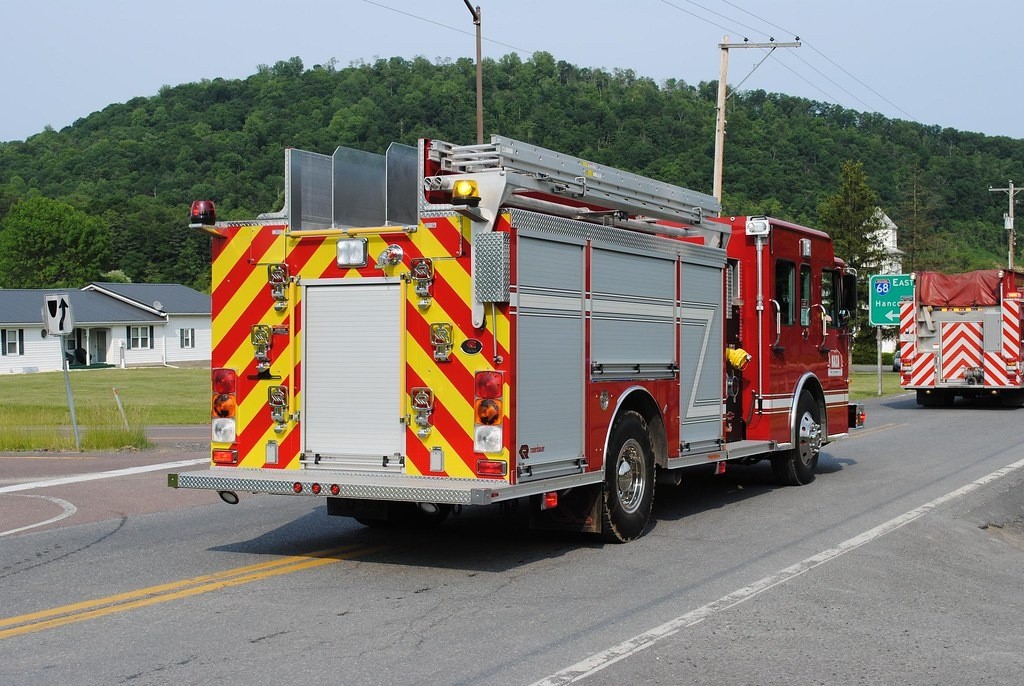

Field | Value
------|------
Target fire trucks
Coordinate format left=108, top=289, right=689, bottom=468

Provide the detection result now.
left=163, top=134, right=867, bottom=544
left=899, top=269, right=1023, bottom=410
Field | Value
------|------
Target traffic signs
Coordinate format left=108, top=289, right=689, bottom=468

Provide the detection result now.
left=869, top=274, right=914, bottom=327
